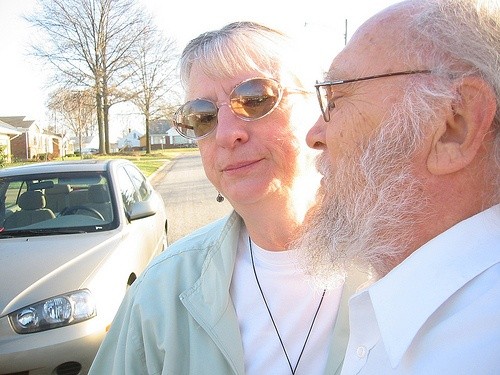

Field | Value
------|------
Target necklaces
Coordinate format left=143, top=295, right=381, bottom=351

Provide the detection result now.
left=248, top=236, right=327, bottom=375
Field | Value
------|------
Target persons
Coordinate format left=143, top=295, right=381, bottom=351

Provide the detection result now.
left=302, top=0, right=500, bottom=375
left=86, top=19, right=380, bottom=375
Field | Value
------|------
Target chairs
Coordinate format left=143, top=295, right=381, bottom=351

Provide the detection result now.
left=4, top=191, right=55, bottom=230
left=73, top=183, right=113, bottom=221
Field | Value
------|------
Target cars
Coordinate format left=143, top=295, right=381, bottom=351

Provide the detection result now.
left=0, top=158, right=168, bottom=375
left=74, top=148, right=89, bottom=157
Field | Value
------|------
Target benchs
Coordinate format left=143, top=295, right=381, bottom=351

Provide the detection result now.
left=45, top=183, right=111, bottom=216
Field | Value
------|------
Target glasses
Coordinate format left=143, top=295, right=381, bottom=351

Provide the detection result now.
left=314, top=70, right=459, bottom=122
left=173, top=77, right=315, bottom=141
